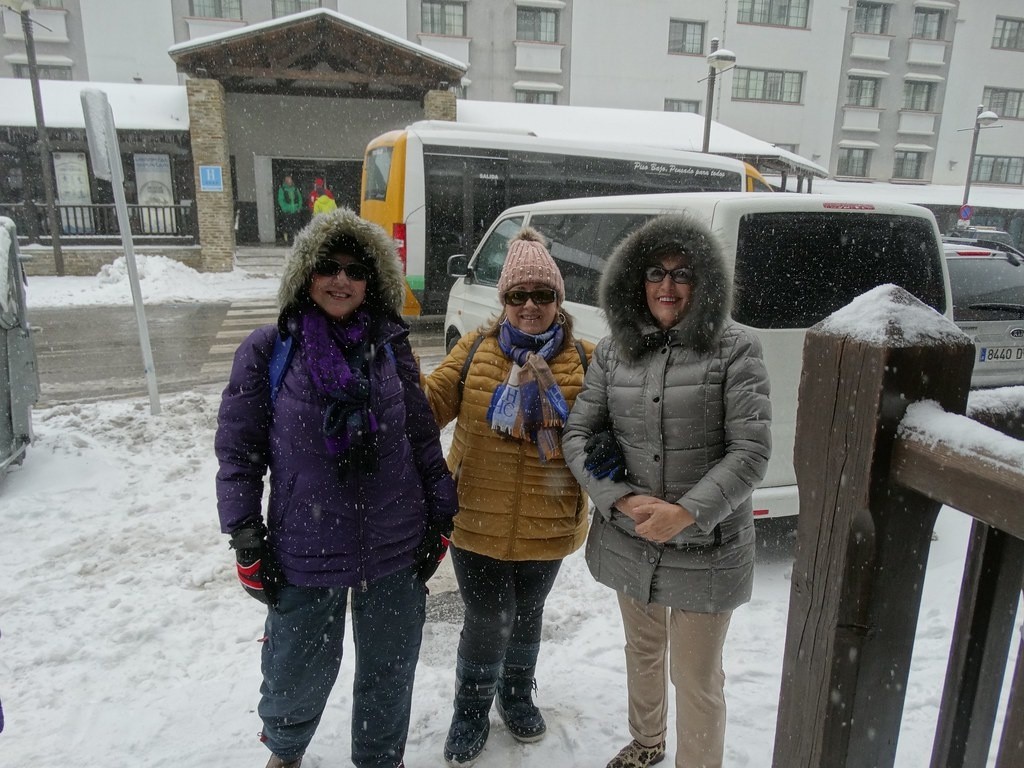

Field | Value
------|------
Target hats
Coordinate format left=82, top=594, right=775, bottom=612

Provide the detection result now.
left=497, top=227, right=565, bottom=308
left=324, top=234, right=375, bottom=267
left=315, top=179, right=323, bottom=185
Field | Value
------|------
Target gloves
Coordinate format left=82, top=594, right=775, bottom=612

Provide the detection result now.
left=584, top=434, right=626, bottom=481
left=414, top=519, right=454, bottom=582
left=229, top=516, right=283, bottom=604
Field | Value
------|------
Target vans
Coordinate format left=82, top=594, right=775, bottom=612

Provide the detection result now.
left=443, top=191, right=954, bottom=518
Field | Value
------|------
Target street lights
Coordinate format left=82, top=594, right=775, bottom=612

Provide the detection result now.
left=701, top=49, right=736, bottom=154
left=962, top=110, right=999, bottom=219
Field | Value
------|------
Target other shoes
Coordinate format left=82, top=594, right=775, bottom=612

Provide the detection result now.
left=266, top=753, right=303, bottom=768
left=606, top=740, right=666, bottom=768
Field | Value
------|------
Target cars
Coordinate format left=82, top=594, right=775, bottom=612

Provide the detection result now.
left=940, top=238, right=1024, bottom=419
left=942, top=226, right=1015, bottom=249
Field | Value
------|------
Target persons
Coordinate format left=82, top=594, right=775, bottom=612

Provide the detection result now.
left=278, top=175, right=302, bottom=243
left=308, top=177, right=334, bottom=215
left=424, top=225, right=595, bottom=768
left=314, top=188, right=338, bottom=218
left=561, top=207, right=772, bottom=768
left=215, top=206, right=459, bottom=768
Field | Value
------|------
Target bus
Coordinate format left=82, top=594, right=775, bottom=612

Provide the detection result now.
left=360, top=119, right=777, bottom=325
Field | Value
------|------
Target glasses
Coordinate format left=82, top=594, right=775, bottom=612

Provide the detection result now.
left=502, top=288, right=556, bottom=306
left=644, top=265, right=695, bottom=284
left=314, top=259, right=370, bottom=281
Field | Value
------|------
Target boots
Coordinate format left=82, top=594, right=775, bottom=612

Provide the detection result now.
left=499, top=649, right=547, bottom=743
left=445, top=647, right=508, bottom=768
